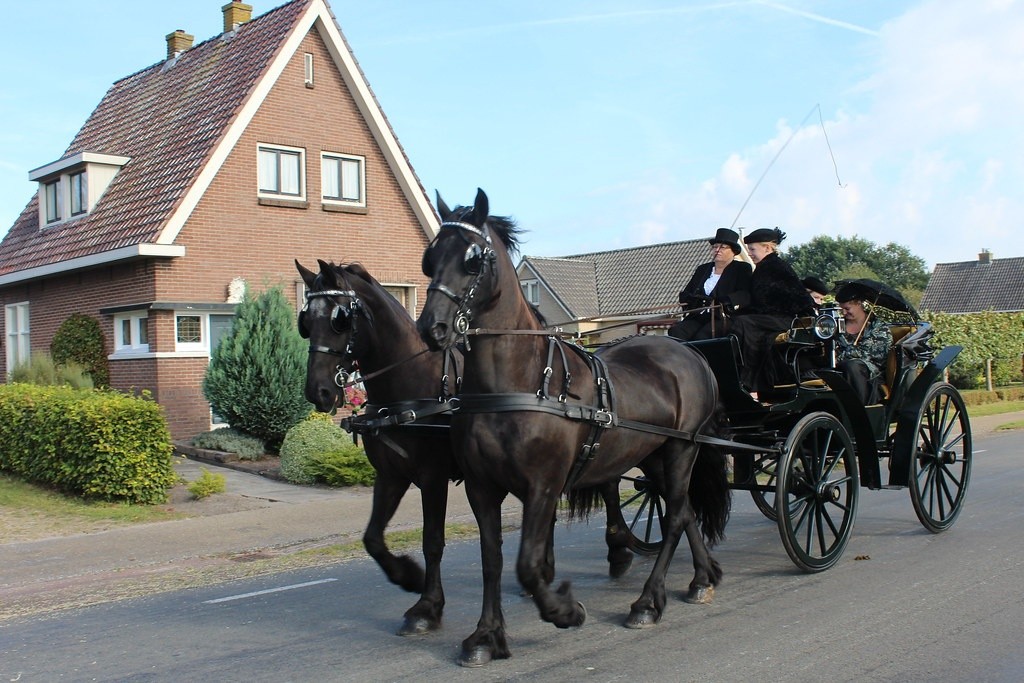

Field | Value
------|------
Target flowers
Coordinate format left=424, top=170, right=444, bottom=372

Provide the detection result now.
left=345, top=387, right=366, bottom=411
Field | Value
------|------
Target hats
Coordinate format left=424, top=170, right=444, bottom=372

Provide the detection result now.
left=836, top=284, right=865, bottom=303
left=709, top=228, right=741, bottom=255
left=801, top=276, right=829, bottom=296
left=744, top=227, right=787, bottom=245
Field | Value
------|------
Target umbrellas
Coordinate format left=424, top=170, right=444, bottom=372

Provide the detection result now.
left=830, top=278, right=921, bottom=346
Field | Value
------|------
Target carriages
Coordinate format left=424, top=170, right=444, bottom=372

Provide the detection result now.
left=294, top=186, right=973, bottom=669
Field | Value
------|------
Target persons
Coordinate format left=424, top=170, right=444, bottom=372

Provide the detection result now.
left=801, top=277, right=830, bottom=319
left=824, top=287, right=894, bottom=404
left=665, top=225, right=820, bottom=408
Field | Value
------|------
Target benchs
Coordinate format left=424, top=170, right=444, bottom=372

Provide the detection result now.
left=774, top=316, right=932, bottom=401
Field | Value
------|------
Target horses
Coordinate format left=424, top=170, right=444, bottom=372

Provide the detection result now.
left=415, top=187, right=732, bottom=671
left=293, top=258, right=633, bottom=635
